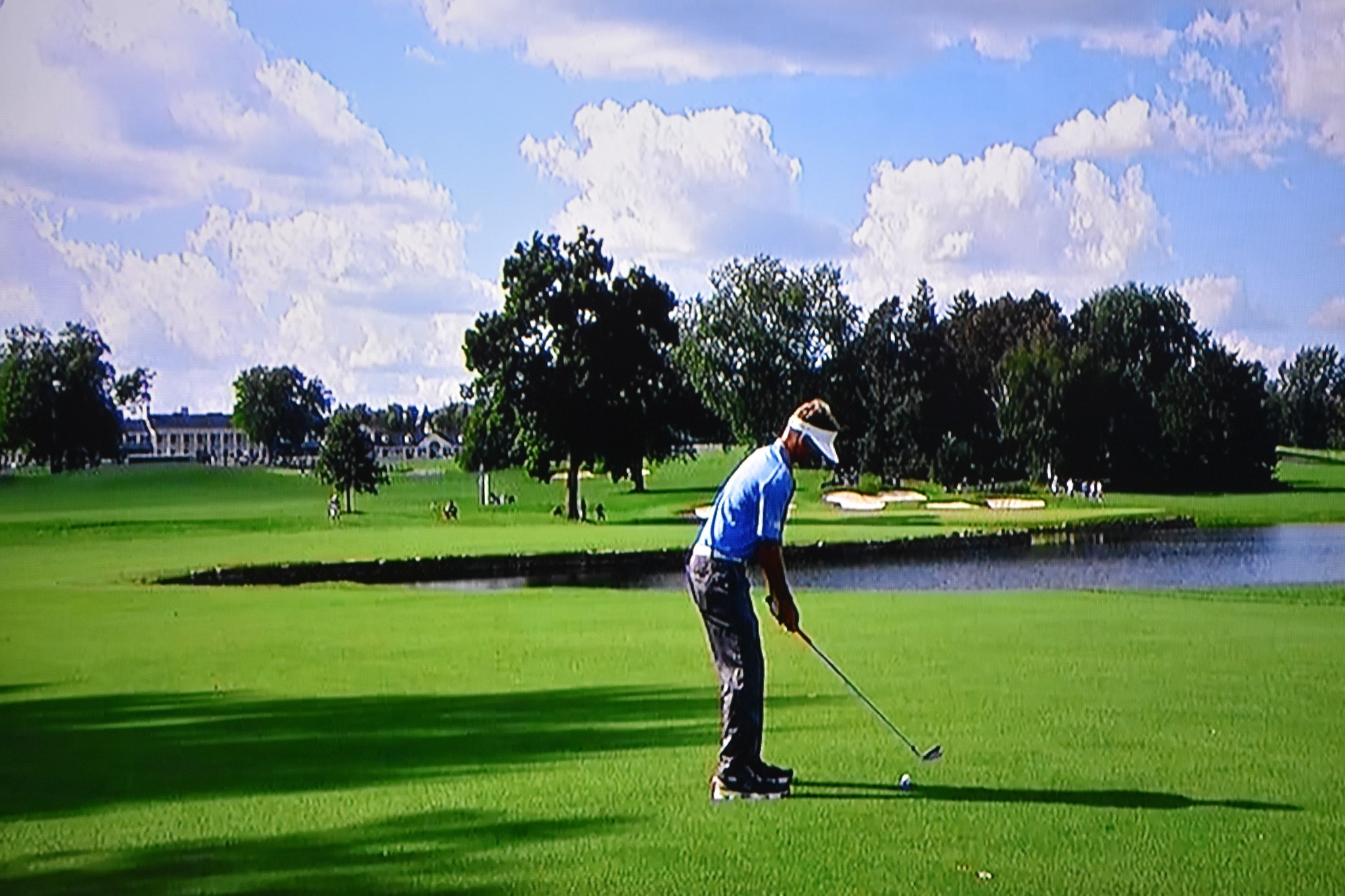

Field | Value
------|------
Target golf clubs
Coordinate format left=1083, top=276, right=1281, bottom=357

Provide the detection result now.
left=764, top=593, right=944, bottom=761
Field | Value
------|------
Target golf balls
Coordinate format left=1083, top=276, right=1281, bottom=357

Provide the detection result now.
left=898, top=774, right=911, bottom=786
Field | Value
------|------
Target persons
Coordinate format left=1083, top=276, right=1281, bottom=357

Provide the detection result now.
left=327, top=490, right=607, bottom=521
left=686, top=398, right=841, bottom=797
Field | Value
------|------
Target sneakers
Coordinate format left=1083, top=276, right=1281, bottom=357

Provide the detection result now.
left=709, top=776, right=790, bottom=801
left=750, top=761, right=794, bottom=785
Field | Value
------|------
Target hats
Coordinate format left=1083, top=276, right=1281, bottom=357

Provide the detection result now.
left=788, top=416, right=839, bottom=463
